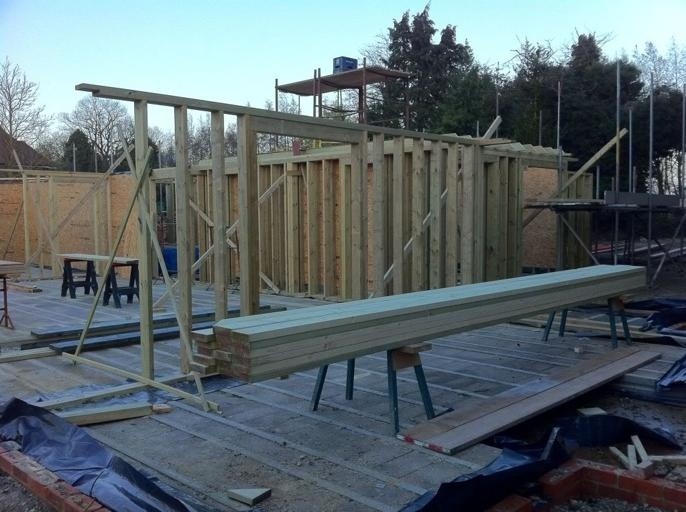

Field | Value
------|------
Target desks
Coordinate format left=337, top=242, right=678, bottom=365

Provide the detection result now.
left=62, top=253, right=141, bottom=307
left=1, top=275, right=17, bottom=328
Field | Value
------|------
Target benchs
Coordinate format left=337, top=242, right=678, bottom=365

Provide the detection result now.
left=308, top=339, right=436, bottom=431
left=541, top=292, right=636, bottom=350
